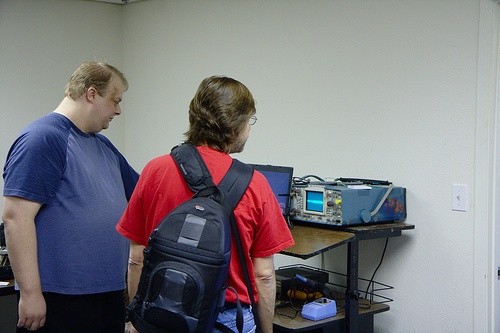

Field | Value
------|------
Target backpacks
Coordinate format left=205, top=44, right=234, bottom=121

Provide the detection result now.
left=124, top=142, right=257, bottom=333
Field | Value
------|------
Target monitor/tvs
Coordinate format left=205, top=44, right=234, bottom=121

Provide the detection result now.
left=246, top=163, right=294, bottom=218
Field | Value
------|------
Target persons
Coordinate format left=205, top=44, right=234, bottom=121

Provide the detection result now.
left=116, top=76, right=296, bottom=333
left=2, top=60, right=142, bottom=333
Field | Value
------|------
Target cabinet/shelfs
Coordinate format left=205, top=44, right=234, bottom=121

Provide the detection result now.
left=273, top=221, right=415, bottom=333
